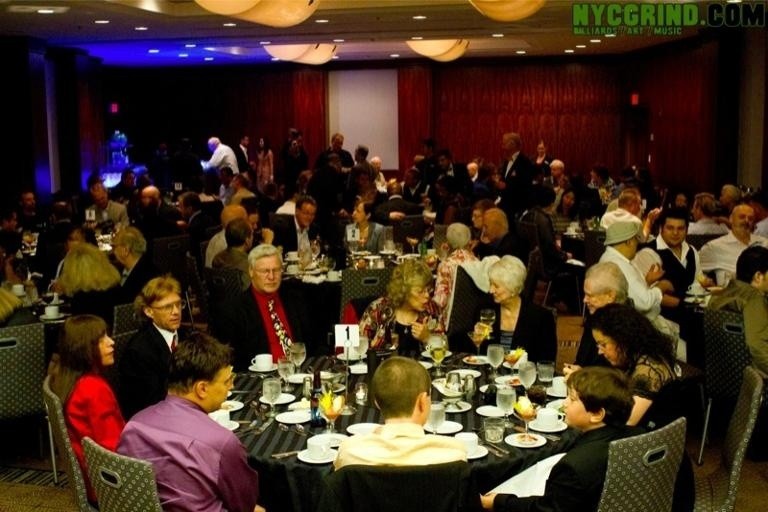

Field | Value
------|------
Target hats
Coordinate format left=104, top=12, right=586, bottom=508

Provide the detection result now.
left=603, top=221, right=640, bottom=246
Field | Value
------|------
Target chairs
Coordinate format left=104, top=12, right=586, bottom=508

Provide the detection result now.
left=112, top=302, right=145, bottom=366
left=82, top=435, right=161, bottom=512
left=445, top=266, right=489, bottom=337
left=0, top=323, right=60, bottom=487
left=596, top=417, right=687, bottom=511
left=432, top=224, right=450, bottom=249
left=43, top=365, right=96, bottom=511
left=203, top=265, right=245, bottom=331
left=693, top=366, right=762, bottom=512
left=583, top=230, right=606, bottom=267
left=393, top=215, right=434, bottom=253
left=518, top=221, right=582, bottom=317
left=338, top=269, right=397, bottom=324
left=152, top=234, right=196, bottom=331
left=696, top=307, right=751, bottom=466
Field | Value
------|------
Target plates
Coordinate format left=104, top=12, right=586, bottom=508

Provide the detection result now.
left=336, top=353, right=368, bottom=361
left=289, top=373, right=315, bottom=384
left=334, top=382, right=347, bottom=393
left=528, top=419, right=567, bottom=433
left=424, top=420, right=463, bottom=434
left=448, top=368, right=482, bottom=380
left=546, top=399, right=566, bottom=415
left=40, top=313, right=64, bottom=319
left=431, top=378, right=467, bottom=397
left=274, top=412, right=314, bottom=425
left=686, top=290, right=706, bottom=296
left=420, top=349, right=453, bottom=358
left=222, top=400, right=244, bottom=413
left=546, top=387, right=569, bottom=399
left=494, top=375, right=525, bottom=387
left=311, top=433, right=348, bottom=448
left=297, top=448, right=339, bottom=464
left=463, top=355, right=490, bottom=365
left=284, top=257, right=301, bottom=262
left=248, top=364, right=280, bottom=373
left=220, top=420, right=240, bottom=432
left=346, top=422, right=380, bottom=435
left=476, top=405, right=511, bottom=417
left=417, top=361, right=434, bottom=370
left=502, top=360, right=522, bottom=371
left=324, top=278, right=341, bottom=282
left=444, top=401, right=472, bottom=414
left=259, top=393, right=296, bottom=405
left=504, top=432, right=547, bottom=448
left=465, top=446, right=489, bottom=461
left=684, top=297, right=703, bottom=304
left=479, top=384, right=506, bottom=393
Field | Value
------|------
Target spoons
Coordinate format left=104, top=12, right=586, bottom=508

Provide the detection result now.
left=259, top=404, right=268, bottom=420
left=278, top=423, right=306, bottom=437
left=250, top=401, right=259, bottom=417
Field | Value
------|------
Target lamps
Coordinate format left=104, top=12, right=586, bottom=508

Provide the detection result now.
left=228, top=0, right=321, bottom=27
left=193, top=0, right=257, bottom=16
left=429, top=39, right=473, bottom=62
left=406, top=39, right=457, bottom=55
left=469, top=0, right=546, bottom=22
left=292, top=43, right=341, bottom=65
left=262, top=43, right=310, bottom=60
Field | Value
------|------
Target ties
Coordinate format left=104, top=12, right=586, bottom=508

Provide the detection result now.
left=171, top=335, right=178, bottom=351
left=266, top=299, right=294, bottom=359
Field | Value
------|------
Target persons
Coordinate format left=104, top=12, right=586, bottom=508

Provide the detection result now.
left=454, top=255, right=558, bottom=366
left=566, top=163, right=605, bottom=221
left=472, top=209, right=523, bottom=261
left=0, top=227, right=28, bottom=287
left=35, top=200, right=98, bottom=258
left=432, top=223, right=479, bottom=332
left=201, top=137, right=240, bottom=178
left=687, top=192, right=729, bottom=234
left=134, top=184, right=184, bottom=240
left=78, top=176, right=131, bottom=240
left=1, top=207, right=22, bottom=252
left=707, top=245, right=768, bottom=381
left=589, top=164, right=617, bottom=197
left=747, top=192, right=768, bottom=239
left=521, top=185, right=583, bottom=317
left=16, top=191, right=50, bottom=238
left=341, top=198, right=384, bottom=267
left=530, top=139, right=553, bottom=173
left=481, top=366, right=695, bottom=512
left=578, top=304, right=683, bottom=426
left=630, top=207, right=701, bottom=303
left=598, top=211, right=680, bottom=338
left=698, top=204, right=768, bottom=290
left=212, top=217, right=254, bottom=291
left=573, top=262, right=634, bottom=366
left=112, top=227, right=176, bottom=304
left=358, top=259, right=443, bottom=357
left=176, top=191, right=222, bottom=242
left=471, top=199, right=496, bottom=229
left=241, top=203, right=264, bottom=249
left=543, top=158, right=572, bottom=188
left=53, top=243, right=127, bottom=335
left=491, top=132, right=533, bottom=233
left=270, top=195, right=335, bottom=259
left=334, top=356, right=467, bottom=472
left=108, top=169, right=138, bottom=203
left=714, top=184, right=740, bottom=231
left=548, top=187, right=583, bottom=234
left=630, top=206, right=715, bottom=367
left=114, top=333, right=266, bottom=512
left=213, top=167, right=234, bottom=204
left=600, top=188, right=663, bottom=245
left=204, top=204, right=248, bottom=268
left=51, top=315, right=126, bottom=512
left=238, top=126, right=500, bottom=210
left=226, top=244, right=331, bottom=372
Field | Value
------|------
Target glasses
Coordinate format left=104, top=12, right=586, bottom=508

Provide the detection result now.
left=149, top=299, right=188, bottom=314
left=415, top=287, right=435, bottom=295
left=254, top=266, right=284, bottom=274
left=597, top=341, right=610, bottom=350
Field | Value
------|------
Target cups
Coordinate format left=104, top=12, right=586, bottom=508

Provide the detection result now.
left=44, top=306, right=58, bottom=317
left=12, top=284, right=24, bottom=295
left=251, top=354, right=273, bottom=371
left=484, top=418, right=505, bottom=443
left=488, top=345, right=504, bottom=379
left=430, top=339, right=445, bottom=377
left=175, top=183, right=182, bottom=190
left=302, top=376, right=312, bottom=400
left=364, top=255, right=380, bottom=270
left=513, top=404, right=538, bottom=444
left=278, top=359, right=295, bottom=393
left=355, top=383, right=367, bottom=400
left=427, top=401, right=444, bottom=436
left=284, top=252, right=299, bottom=258
left=326, top=271, right=338, bottom=280
left=538, top=408, right=562, bottom=427
left=290, top=343, right=307, bottom=373
left=262, top=378, right=282, bottom=418
left=354, top=337, right=369, bottom=364
left=503, top=350, right=524, bottom=384
left=537, top=361, right=553, bottom=382
left=479, top=308, right=495, bottom=340
left=85, top=209, right=95, bottom=221
left=454, top=432, right=478, bottom=454
left=553, top=377, right=568, bottom=395
left=495, top=388, right=516, bottom=428
left=379, top=250, right=395, bottom=262
left=518, top=362, right=537, bottom=393
left=321, top=395, right=345, bottom=436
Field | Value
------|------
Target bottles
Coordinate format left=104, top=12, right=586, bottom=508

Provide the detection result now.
left=383, top=322, right=394, bottom=359
left=310, top=369, right=324, bottom=422
